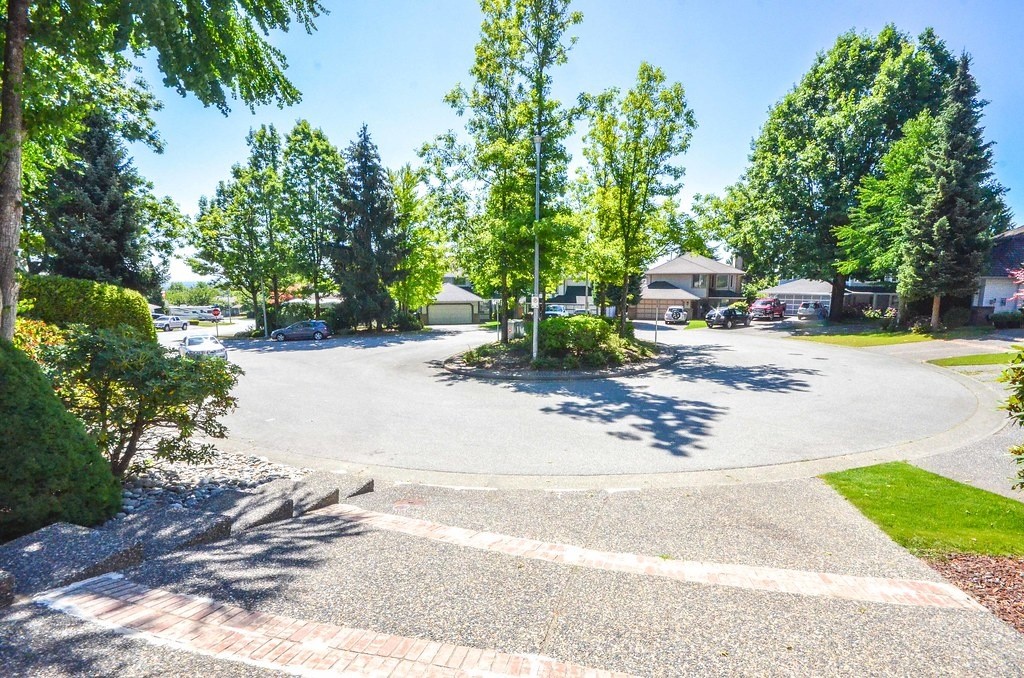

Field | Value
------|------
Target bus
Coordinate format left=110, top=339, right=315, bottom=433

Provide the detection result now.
left=169, top=304, right=224, bottom=321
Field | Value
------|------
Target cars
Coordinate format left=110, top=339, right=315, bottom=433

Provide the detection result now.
left=152, top=313, right=166, bottom=322
left=574, top=309, right=590, bottom=316
left=271, top=318, right=332, bottom=342
left=153, top=316, right=190, bottom=332
left=178, top=334, right=228, bottom=362
left=705, top=306, right=753, bottom=329
left=797, top=301, right=829, bottom=321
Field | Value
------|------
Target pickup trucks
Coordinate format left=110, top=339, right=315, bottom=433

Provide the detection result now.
left=748, top=298, right=786, bottom=321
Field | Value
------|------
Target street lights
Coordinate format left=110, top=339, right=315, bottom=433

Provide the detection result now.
left=221, top=236, right=269, bottom=340
left=533, top=134, right=542, bottom=362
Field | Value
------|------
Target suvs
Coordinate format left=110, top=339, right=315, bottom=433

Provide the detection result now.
left=664, top=305, right=688, bottom=325
left=543, top=304, right=569, bottom=320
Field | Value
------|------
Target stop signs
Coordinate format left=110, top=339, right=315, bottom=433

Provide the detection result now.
left=212, top=309, right=220, bottom=316
